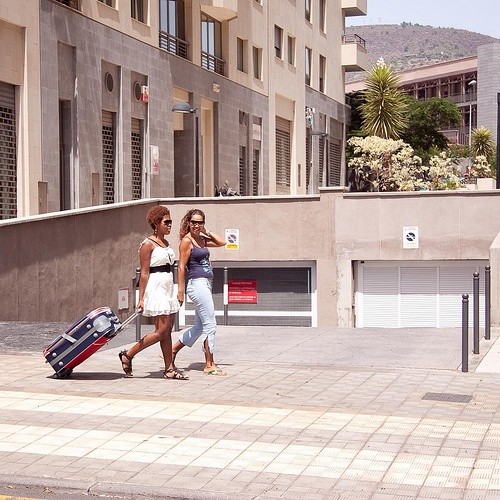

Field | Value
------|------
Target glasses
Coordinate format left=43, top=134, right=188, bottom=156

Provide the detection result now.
left=189, top=220, right=205, bottom=226
left=161, top=219, right=172, bottom=225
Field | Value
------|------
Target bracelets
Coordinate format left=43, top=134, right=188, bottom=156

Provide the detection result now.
left=207, top=230, right=210, bottom=237
left=178, top=300, right=183, bottom=302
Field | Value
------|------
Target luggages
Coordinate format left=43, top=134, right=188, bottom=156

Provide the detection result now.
left=43, top=306, right=143, bottom=379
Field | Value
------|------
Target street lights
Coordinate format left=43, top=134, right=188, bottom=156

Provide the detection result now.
left=308, top=129, right=330, bottom=187
left=467, top=79, right=476, bottom=151
left=171, top=101, right=200, bottom=197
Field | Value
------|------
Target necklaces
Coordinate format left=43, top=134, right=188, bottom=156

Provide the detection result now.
left=149, top=238, right=164, bottom=248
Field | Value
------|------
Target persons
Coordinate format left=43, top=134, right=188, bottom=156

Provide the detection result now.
left=119, top=206, right=188, bottom=379
left=218, top=179, right=243, bottom=196
left=158, top=210, right=225, bottom=376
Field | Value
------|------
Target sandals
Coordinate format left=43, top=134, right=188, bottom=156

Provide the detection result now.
left=119, top=350, right=134, bottom=378
left=163, top=369, right=189, bottom=380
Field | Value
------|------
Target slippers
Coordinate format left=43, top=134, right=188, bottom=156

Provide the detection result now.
left=203, top=367, right=227, bottom=376
